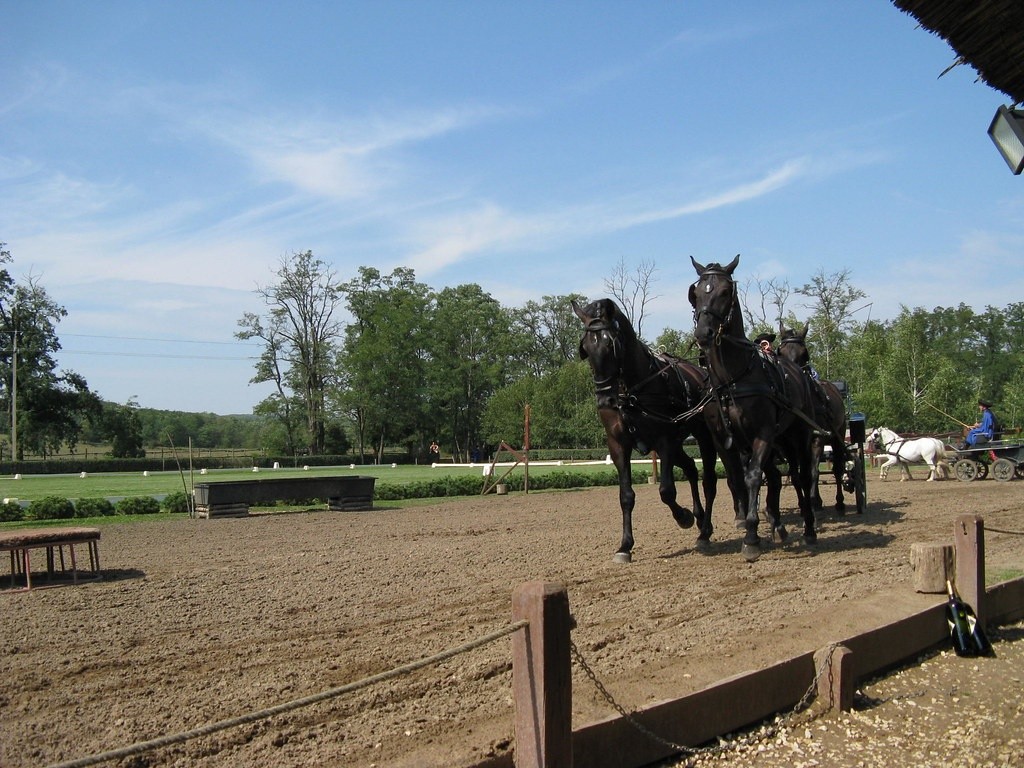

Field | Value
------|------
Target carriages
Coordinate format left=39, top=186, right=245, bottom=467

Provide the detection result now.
left=866, top=426, right=1024, bottom=483
left=572, top=255, right=865, bottom=562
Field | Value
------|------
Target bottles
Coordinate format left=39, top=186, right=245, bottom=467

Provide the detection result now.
left=945, top=580, right=972, bottom=655
left=952, top=585, right=991, bottom=654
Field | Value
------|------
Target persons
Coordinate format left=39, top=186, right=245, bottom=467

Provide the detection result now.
left=958, top=399, right=997, bottom=450
left=754, top=332, right=819, bottom=380
left=430, top=442, right=439, bottom=453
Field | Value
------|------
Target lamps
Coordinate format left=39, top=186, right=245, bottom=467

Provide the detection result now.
left=987, top=103, right=1024, bottom=176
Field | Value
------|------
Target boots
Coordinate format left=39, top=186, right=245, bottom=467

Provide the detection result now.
left=958, top=441, right=970, bottom=450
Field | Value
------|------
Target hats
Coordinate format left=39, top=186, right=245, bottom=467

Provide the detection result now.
left=978, top=399, right=993, bottom=407
left=754, top=333, right=776, bottom=344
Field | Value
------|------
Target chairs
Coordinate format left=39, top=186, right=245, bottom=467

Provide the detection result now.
left=972, top=423, right=1005, bottom=447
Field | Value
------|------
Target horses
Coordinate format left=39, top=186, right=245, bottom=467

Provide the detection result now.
left=687, top=253, right=847, bottom=560
left=570, top=299, right=748, bottom=564
left=867, top=426, right=950, bottom=482
